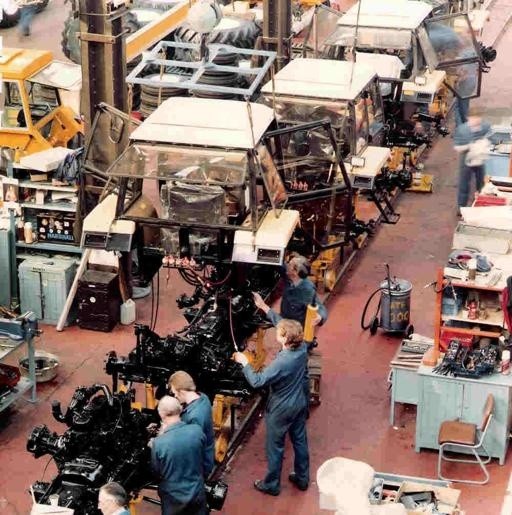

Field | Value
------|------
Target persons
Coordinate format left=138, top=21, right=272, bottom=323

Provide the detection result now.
left=428, top=21, right=491, bottom=216
left=234, top=290, right=310, bottom=496
left=97, top=482, right=130, bottom=515
left=276, top=251, right=328, bottom=352
left=15, top=0, right=41, bottom=36
left=150, top=395, right=208, bottom=515
left=146, top=370, right=215, bottom=479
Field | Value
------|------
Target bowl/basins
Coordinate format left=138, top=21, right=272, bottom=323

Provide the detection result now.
left=18, top=357, right=59, bottom=383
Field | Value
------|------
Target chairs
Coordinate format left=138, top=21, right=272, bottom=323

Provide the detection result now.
left=434, top=393, right=498, bottom=485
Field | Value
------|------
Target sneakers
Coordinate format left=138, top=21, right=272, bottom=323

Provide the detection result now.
left=254, top=479, right=279, bottom=496
left=289, top=471, right=308, bottom=490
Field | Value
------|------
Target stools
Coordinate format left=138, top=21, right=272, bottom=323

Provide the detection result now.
left=307, top=350, right=322, bottom=407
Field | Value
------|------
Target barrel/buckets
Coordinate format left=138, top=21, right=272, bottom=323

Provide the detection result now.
left=119, top=298, right=137, bottom=326
left=380, top=279, right=413, bottom=330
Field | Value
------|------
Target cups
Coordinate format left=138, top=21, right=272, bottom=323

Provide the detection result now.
left=52, top=179, right=57, bottom=187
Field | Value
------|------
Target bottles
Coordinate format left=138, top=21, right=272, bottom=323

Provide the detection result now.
left=501, top=350, right=510, bottom=375
left=18, top=221, right=25, bottom=243
left=24, top=222, right=33, bottom=244
left=467, top=302, right=486, bottom=320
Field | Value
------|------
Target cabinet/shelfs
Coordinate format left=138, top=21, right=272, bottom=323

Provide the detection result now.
left=433, top=264, right=512, bottom=361
left=413, top=345, right=512, bottom=467
left=387, top=339, right=431, bottom=426
left=1, top=327, right=44, bottom=416
left=6, top=166, right=85, bottom=257
left=17, top=257, right=78, bottom=327
